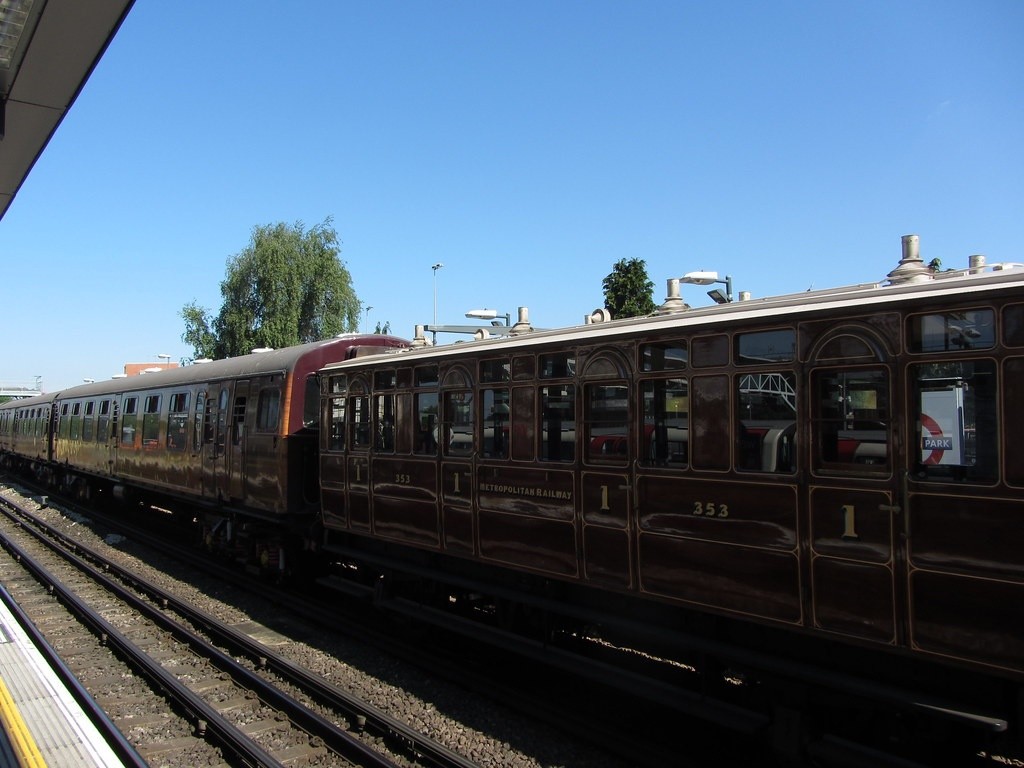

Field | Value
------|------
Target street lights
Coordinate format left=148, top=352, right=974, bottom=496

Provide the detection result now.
left=837, top=385, right=854, bottom=431
left=465, top=309, right=510, bottom=327
left=949, top=325, right=982, bottom=376
left=432, top=263, right=444, bottom=346
left=679, top=272, right=733, bottom=303
left=158, top=354, right=170, bottom=369
left=747, top=405, right=752, bottom=420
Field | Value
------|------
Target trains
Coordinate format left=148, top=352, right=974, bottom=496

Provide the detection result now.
left=0, top=235, right=1024, bottom=733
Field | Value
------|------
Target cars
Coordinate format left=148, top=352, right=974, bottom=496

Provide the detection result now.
left=964, top=423, right=976, bottom=434
left=143, top=439, right=158, bottom=445
